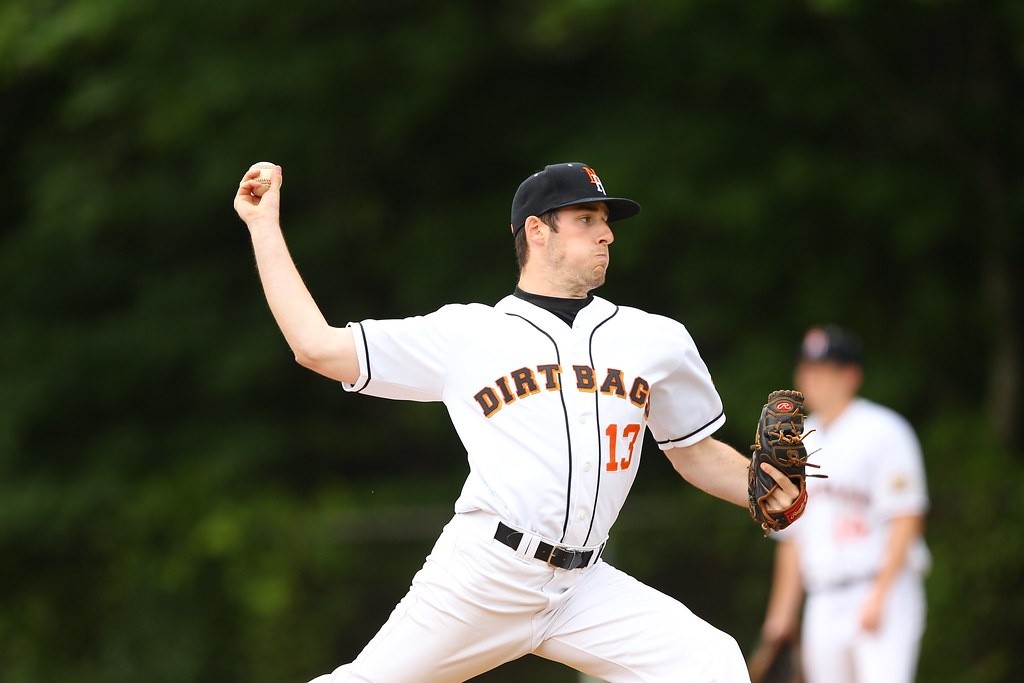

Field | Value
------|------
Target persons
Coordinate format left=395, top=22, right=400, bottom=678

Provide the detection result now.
left=234, top=162, right=807, bottom=683
left=747, top=320, right=933, bottom=683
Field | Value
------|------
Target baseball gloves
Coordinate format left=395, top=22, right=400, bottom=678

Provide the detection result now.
left=746, top=389, right=808, bottom=531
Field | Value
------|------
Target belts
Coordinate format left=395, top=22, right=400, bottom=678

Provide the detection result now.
left=494, top=523, right=608, bottom=571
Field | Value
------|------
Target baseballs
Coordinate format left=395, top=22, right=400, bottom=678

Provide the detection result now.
left=249, top=161, right=282, bottom=198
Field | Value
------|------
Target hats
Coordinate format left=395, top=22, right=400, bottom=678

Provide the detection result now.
left=510, top=163, right=639, bottom=235
left=793, top=324, right=871, bottom=368
